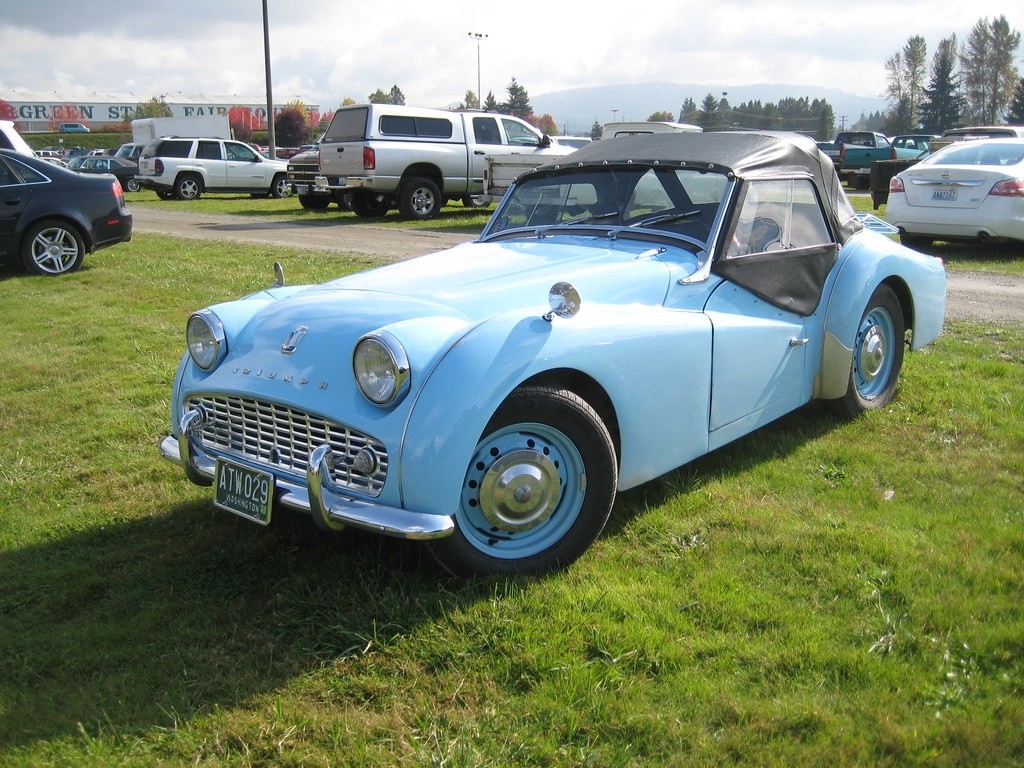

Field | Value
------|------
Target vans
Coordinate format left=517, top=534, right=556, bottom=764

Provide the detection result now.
left=59, top=123, right=91, bottom=134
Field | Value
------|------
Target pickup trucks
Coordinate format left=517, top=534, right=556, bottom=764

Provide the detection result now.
left=869, top=126, right=1024, bottom=210
left=815, top=131, right=891, bottom=181
left=313, top=104, right=578, bottom=221
left=838, top=134, right=942, bottom=190
left=478, top=121, right=704, bottom=224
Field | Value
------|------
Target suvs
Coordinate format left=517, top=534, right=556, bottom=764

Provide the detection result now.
left=134, top=136, right=297, bottom=198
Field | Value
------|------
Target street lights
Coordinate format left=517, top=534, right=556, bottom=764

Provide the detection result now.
left=611, top=109, right=619, bottom=122
left=734, top=123, right=739, bottom=131
left=778, top=116, right=783, bottom=128
left=468, top=32, right=489, bottom=109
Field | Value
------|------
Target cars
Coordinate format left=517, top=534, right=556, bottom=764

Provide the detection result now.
left=286, top=131, right=354, bottom=212
left=0, top=147, right=132, bottom=277
left=886, top=138, right=1024, bottom=252
left=0, top=120, right=36, bottom=160
left=159, top=129, right=948, bottom=586
left=247, top=143, right=317, bottom=159
left=511, top=136, right=592, bottom=150
left=600, top=122, right=704, bottom=141
left=32, top=143, right=145, bottom=194
